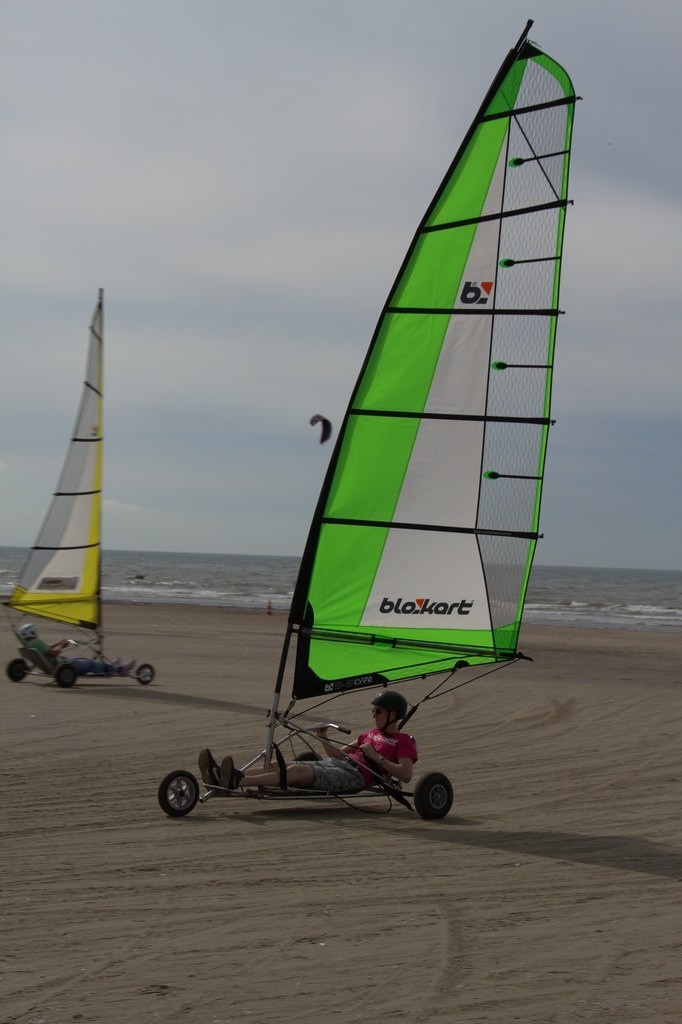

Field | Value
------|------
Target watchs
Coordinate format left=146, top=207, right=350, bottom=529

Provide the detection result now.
left=378, top=755, right=385, bottom=764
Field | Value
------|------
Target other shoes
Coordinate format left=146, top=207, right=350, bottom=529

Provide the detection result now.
left=110, top=658, right=136, bottom=676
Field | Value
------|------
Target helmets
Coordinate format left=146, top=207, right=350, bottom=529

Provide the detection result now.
left=20, top=623, right=38, bottom=640
left=371, top=691, right=407, bottom=720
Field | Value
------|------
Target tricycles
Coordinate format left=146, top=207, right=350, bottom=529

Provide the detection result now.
left=158, top=11, right=605, bottom=820
left=0, top=283, right=155, bottom=688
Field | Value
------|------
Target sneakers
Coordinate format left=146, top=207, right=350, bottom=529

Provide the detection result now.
left=198, top=747, right=244, bottom=794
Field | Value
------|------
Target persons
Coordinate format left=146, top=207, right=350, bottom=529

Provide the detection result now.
left=18, top=624, right=137, bottom=682
left=198, top=690, right=419, bottom=795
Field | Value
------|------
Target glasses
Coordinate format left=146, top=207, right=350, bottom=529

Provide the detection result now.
left=372, top=710, right=391, bottom=715
